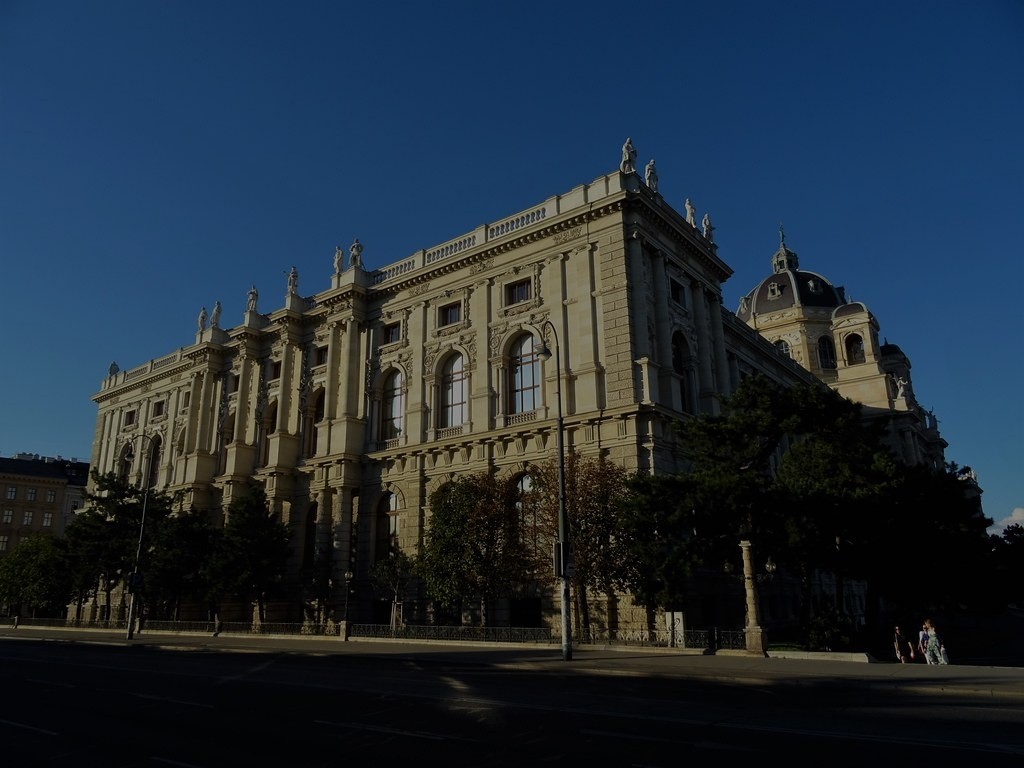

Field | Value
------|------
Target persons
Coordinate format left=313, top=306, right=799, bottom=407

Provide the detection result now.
left=702, top=213, right=712, bottom=238
left=918, top=619, right=950, bottom=665
left=685, top=198, right=697, bottom=227
left=196, top=306, right=207, bottom=331
left=621, top=137, right=634, bottom=173
left=108, top=361, right=119, bottom=378
left=645, top=159, right=657, bottom=192
left=349, top=238, right=363, bottom=268
left=247, top=285, right=257, bottom=310
left=333, top=246, right=343, bottom=272
left=897, top=377, right=908, bottom=398
left=283, top=265, right=298, bottom=295
left=10, top=614, right=18, bottom=629
left=210, top=300, right=222, bottom=327
left=893, top=626, right=914, bottom=664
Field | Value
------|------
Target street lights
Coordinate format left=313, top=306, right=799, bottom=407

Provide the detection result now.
left=536, top=320, right=574, bottom=662
left=339, top=567, right=353, bottom=642
left=125, top=434, right=156, bottom=641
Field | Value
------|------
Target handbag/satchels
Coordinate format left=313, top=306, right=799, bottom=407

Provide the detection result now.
left=896, top=648, right=901, bottom=660
left=940, top=647, right=949, bottom=665
